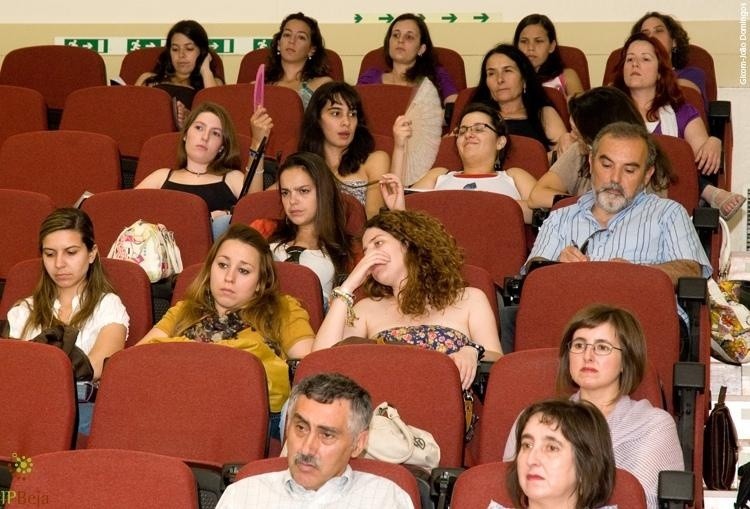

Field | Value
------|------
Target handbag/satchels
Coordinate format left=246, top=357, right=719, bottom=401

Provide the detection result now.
left=28, top=323, right=96, bottom=384
left=701, top=384, right=744, bottom=493
left=105, top=219, right=184, bottom=284
left=708, top=274, right=750, bottom=369
left=360, top=399, right=444, bottom=473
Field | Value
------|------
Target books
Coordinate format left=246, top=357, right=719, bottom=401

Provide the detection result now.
left=109, top=76, right=196, bottom=111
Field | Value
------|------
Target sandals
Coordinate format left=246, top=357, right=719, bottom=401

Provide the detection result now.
left=708, top=187, right=746, bottom=221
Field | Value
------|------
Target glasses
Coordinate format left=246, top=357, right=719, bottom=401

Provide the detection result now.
left=454, top=122, right=500, bottom=136
left=566, top=337, right=624, bottom=356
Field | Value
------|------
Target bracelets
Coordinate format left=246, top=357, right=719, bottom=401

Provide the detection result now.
left=466, top=340, right=485, bottom=359
left=326, top=286, right=359, bottom=328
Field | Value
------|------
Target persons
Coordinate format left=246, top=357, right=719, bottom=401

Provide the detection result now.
left=1, top=206, right=131, bottom=436
left=502, top=302, right=686, bottom=509
left=214, top=371, right=414, bottom=509
left=132, top=14, right=748, bottom=312
left=485, top=400, right=618, bottom=509
left=133, top=223, right=317, bottom=439
left=311, top=205, right=503, bottom=443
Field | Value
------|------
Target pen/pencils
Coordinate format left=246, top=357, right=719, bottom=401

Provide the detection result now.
left=571, top=239, right=580, bottom=248
